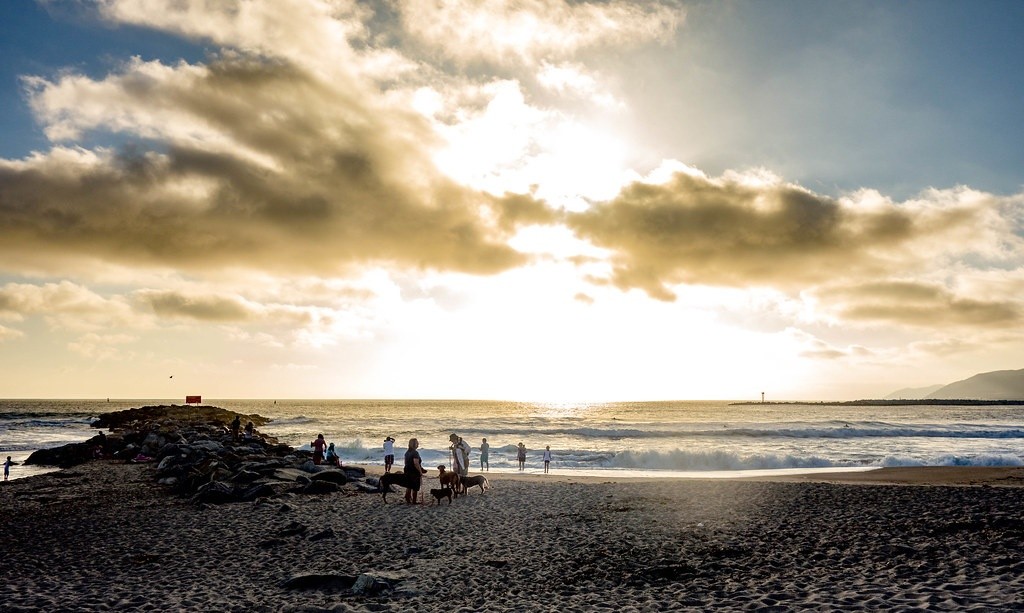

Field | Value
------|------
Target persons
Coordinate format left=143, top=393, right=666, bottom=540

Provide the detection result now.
left=94, top=431, right=106, bottom=460
left=516, top=442, right=527, bottom=471
left=327, top=443, right=339, bottom=466
left=245, top=422, right=254, bottom=446
left=383, top=436, right=395, bottom=473
left=404, top=438, right=427, bottom=504
left=3, top=456, right=19, bottom=479
left=478, top=438, right=489, bottom=471
left=543, top=446, right=552, bottom=473
left=232, top=416, right=240, bottom=442
left=311, top=434, right=327, bottom=465
left=449, top=434, right=471, bottom=495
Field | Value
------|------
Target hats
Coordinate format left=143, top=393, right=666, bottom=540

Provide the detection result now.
left=449, top=434, right=458, bottom=441
left=330, top=443, right=334, bottom=446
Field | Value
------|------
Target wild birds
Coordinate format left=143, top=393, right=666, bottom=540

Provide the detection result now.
left=169, top=375, right=173, bottom=379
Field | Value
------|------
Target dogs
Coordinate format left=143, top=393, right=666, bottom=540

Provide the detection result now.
left=430, top=488, right=453, bottom=507
left=437, top=465, right=488, bottom=499
left=379, top=464, right=422, bottom=505
